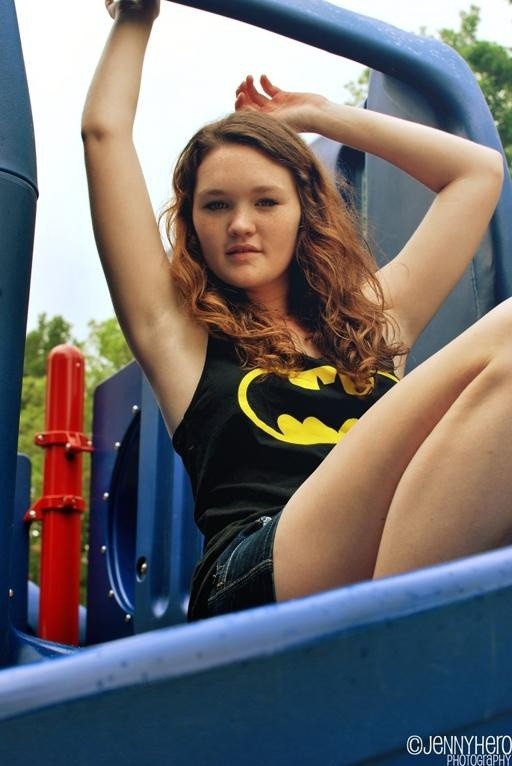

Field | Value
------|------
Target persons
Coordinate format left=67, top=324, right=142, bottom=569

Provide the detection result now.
left=79, top=1, right=512, bottom=622
